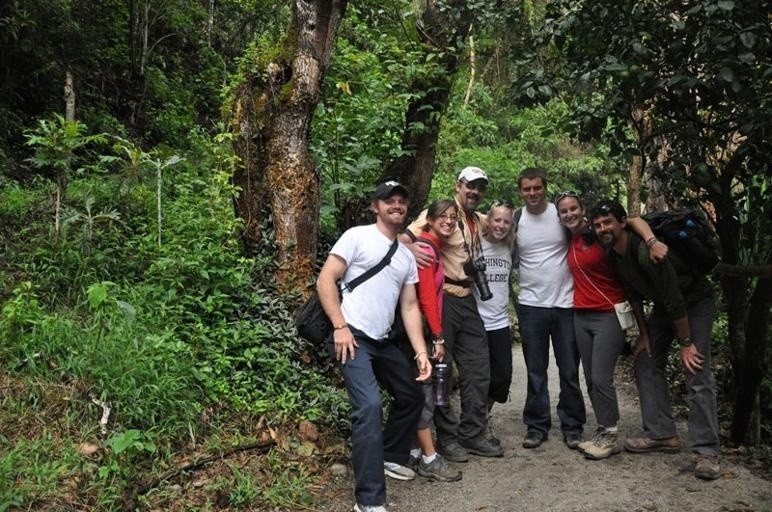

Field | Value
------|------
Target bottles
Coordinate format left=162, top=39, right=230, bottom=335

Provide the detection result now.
left=687, top=219, right=695, bottom=227
left=433, top=363, right=450, bottom=406
left=679, top=230, right=687, bottom=237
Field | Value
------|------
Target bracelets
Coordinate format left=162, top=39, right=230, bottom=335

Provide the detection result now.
left=334, top=323, right=350, bottom=330
left=412, top=350, right=427, bottom=362
left=431, top=337, right=447, bottom=346
left=644, top=235, right=659, bottom=249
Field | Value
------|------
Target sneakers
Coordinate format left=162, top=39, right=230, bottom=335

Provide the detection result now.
left=577, top=428, right=608, bottom=452
left=434, top=437, right=469, bottom=462
left=383, top=459, right=416, bottom=480
left=564, top=431, right=583, bottom=449
left=692, top=454, right=722, bottom=480
left=457, top=428, right=506, bottom=457
left=583, top=431, right=622, bottom=460
left=522, top=431, right=548, bottom=448
left=623, top=436, right=682, bottom=453
left=417, top=454, right=463, bottom=482
left=406, top=451, right=420, bottom=470
left=353, top=502, right=387, bottom=512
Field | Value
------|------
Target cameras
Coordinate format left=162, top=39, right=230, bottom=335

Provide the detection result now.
left=461, top=256, right=493, bottom=302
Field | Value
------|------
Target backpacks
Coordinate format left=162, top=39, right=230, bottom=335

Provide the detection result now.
left=639, top=207, right=719, bottom=275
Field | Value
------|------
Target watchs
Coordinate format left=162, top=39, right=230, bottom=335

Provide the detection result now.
left=678, top=337, right=694, bottom=348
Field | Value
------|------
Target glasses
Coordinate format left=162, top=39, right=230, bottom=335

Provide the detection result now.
left=554, top=190, right=577, bottom=202
left=591, top=205, right=615, bottom=215
left=437, top=214, right=458, bottom=221
left=488, top=199, right=515, bottom=210
left=462, top=180, right=487, bottom=193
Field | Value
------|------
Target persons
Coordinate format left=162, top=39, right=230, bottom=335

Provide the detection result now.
left=413, top=197, right=464, bottom=485
left=472, top=198, right=517, bottom=411
left=553, top=187, right=669, bottom=460
left=396, top=165, right=507, bottom=458
left=315, top=179, right=434, bottom=512
left=512, top=165, right=588, bottom=450
left=589, top=196, right=722, bottom=479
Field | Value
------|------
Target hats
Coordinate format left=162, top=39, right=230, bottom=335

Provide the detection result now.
left=376, top=180, right=409, bottom=201
left=458, top=166, right=489, bottom=184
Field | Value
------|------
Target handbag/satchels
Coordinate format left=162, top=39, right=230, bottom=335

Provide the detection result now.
left=613, top=300, right=636, bottom=331
left=295, top=283, right=344, bottom=348
left=388, top=308, right=432, bottom=365
